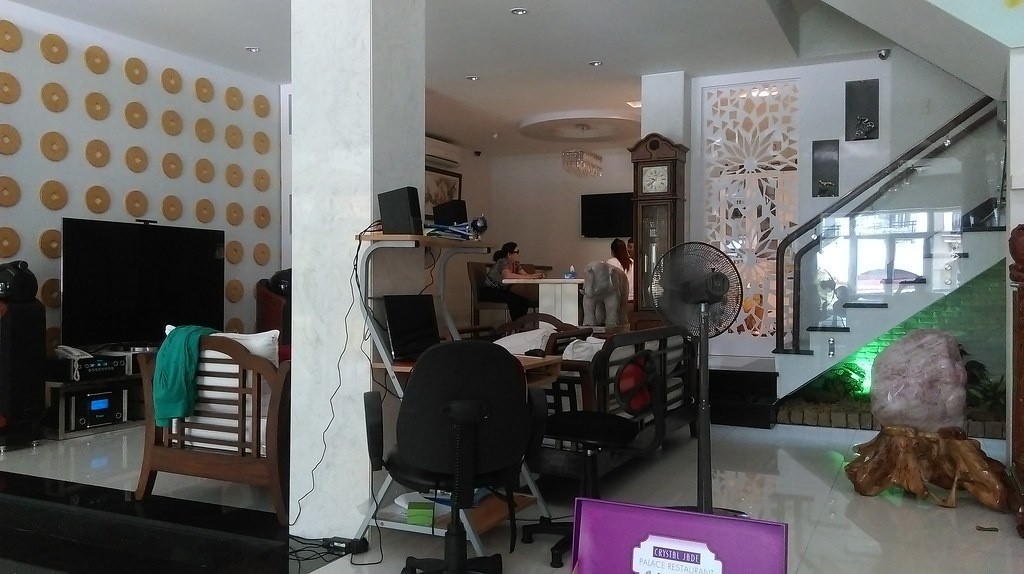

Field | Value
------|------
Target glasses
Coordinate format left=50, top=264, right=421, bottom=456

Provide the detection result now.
left=514, top=250, right=519, bottom=254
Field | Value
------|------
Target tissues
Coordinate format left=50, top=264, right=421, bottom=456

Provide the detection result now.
left=564, top=264, right=577, bottom=279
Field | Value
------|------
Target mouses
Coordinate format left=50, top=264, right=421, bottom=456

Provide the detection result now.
left=525, top=349, right=546, bottom=357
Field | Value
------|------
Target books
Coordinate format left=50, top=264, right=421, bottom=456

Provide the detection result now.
left=429, top=221, right=473, bottom=240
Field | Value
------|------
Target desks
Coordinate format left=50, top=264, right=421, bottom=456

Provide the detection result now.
left=502, top=278, right=585, bottom=330
left=375, top=358, right=571, bottom=441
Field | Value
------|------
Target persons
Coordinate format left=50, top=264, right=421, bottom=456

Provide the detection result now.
left=607, top=238, right=634, bottom=301
left=483, top=242, right=545, bottom=331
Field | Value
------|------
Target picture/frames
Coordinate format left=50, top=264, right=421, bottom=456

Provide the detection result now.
left=421, top=164, right=462, bottom=221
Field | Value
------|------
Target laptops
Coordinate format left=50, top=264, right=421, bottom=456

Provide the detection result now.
left=384, top=295, right=441, bottom=362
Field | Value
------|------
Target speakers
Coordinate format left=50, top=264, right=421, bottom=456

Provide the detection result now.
left=378, top=186, right=424, bottom=236
left=433, top=200, right=470, bottom=241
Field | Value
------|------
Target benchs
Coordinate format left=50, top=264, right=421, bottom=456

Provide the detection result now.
left=501, top=324, right=703, bottom=506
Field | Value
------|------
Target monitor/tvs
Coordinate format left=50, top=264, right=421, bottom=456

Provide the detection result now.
left=580, top=192, right=634, bottom=239
left=59, top=217, right=224, bottom=352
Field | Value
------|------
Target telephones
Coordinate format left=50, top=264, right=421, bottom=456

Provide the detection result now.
left=54, top=346, right=93, bottom=359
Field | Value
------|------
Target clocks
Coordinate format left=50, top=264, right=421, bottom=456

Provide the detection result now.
left=635, top=163, right=672, bottom=193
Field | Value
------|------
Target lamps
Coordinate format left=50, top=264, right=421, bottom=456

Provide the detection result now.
left=561, top=124, right=602, bottom=181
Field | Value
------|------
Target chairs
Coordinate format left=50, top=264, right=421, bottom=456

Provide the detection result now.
left=364, top=339, right=547, bottom=574
left=129, top=339, right=291, bottom=528
left=500, top=325, right=596, bottom=416
left=465, top=260, right=526, bottom=331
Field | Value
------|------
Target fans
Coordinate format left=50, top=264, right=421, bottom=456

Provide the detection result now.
left=648, top=242, right=757, bottom=529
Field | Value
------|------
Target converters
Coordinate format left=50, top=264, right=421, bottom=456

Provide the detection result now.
left=324, top=536, right=369, bottom=554
